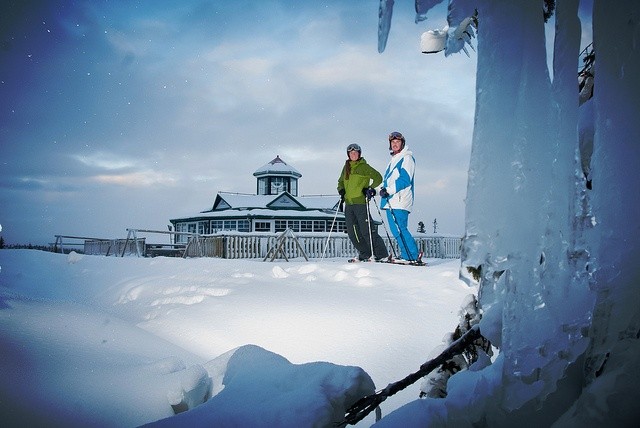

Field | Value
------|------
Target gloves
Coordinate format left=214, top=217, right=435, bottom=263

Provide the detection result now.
left=363, top=185, right=375, bottom=198
left=379, top=188, right=388, bottom=198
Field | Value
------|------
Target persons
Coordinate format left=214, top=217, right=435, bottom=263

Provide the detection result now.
left=337, top=144, right=389, bottom=262
left=381, top=131, right=422, bottom=265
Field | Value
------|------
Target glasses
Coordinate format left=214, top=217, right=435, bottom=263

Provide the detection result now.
left=389, top=133, right=402, bottom=141
left=347, top=145, right=360, bottom=152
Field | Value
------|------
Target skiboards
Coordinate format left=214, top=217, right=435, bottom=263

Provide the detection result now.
left=348, top=257, right=375, bottom=264
left=380, top=254, right=424, bottom=266
